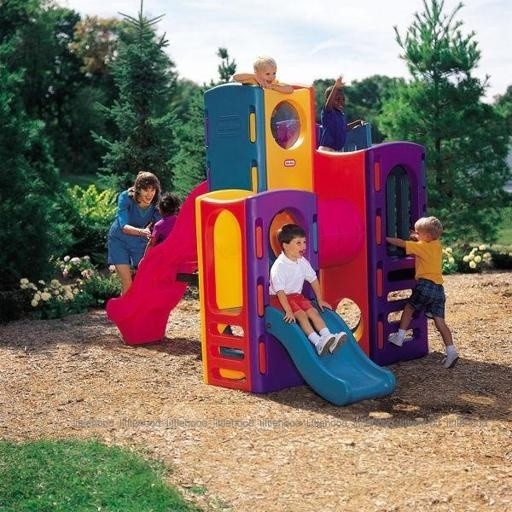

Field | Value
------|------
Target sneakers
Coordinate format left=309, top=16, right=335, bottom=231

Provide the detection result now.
left=314, top=334, right=336, bottom=357
left=388, top=334, right=405, bottom=347
left=444, top=350, right=460, bottom=369
left=328, top=331, right=348, bottom=354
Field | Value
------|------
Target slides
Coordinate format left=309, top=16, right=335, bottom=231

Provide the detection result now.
left=266, top=304, right=397, bottom=407
left=105, top=182, right=208, bottom=345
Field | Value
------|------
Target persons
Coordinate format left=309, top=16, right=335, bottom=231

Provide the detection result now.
left=233, top=57, right=294, bottom=94
left=384, top=215, right=459, bottom=368
left=268, top=223, right=348, bottom=357
left=107, top=172, right=162, bottom=296
left=137, top=193, right=179, bottom=266
left=319, top=74, right=366, bottom=152
